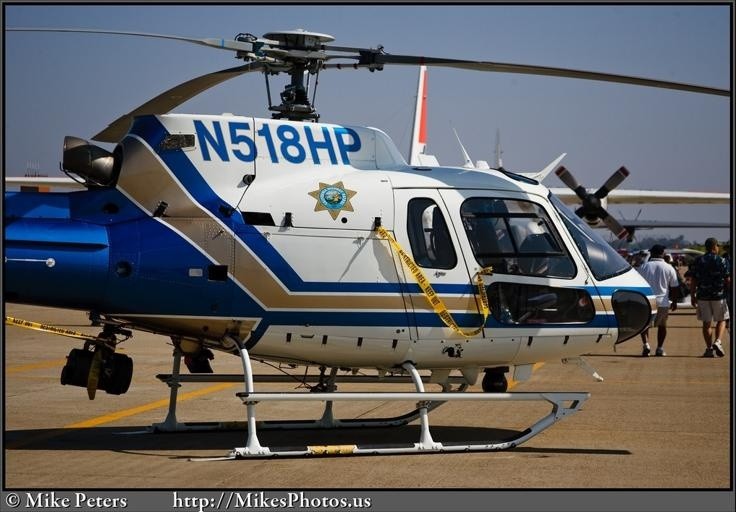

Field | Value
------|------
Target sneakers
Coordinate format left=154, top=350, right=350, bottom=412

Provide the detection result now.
left=655, top=349, right=663, bottom=356
left=643, top=348, right=650, bottom=356
left=704, top=349, right=713, bottom=357
left=712, top=340, right=725, bottom=356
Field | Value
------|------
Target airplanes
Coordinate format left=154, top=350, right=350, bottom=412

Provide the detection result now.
left=3, top=27, right=730, bottom=463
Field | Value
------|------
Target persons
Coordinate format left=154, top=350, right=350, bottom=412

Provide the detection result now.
left=616, top=237, right=730, bottom=357
